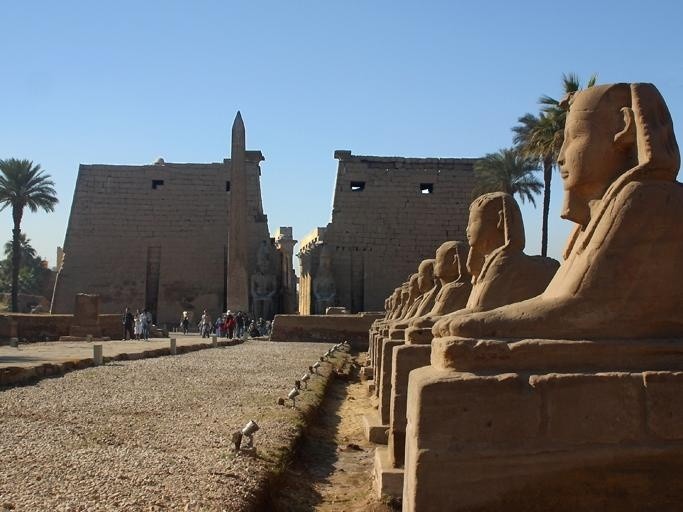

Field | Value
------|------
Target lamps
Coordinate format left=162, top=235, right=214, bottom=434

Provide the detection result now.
left=242, top=418, right=260, bottom=448
left=287, top=340, right=350, bottom=406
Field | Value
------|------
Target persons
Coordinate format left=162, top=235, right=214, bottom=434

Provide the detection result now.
left=180, top=310, right=189, bottom=334
left=197, top=308, right=269, bottom=339
left=122, top=306, right=153, bottom=341
